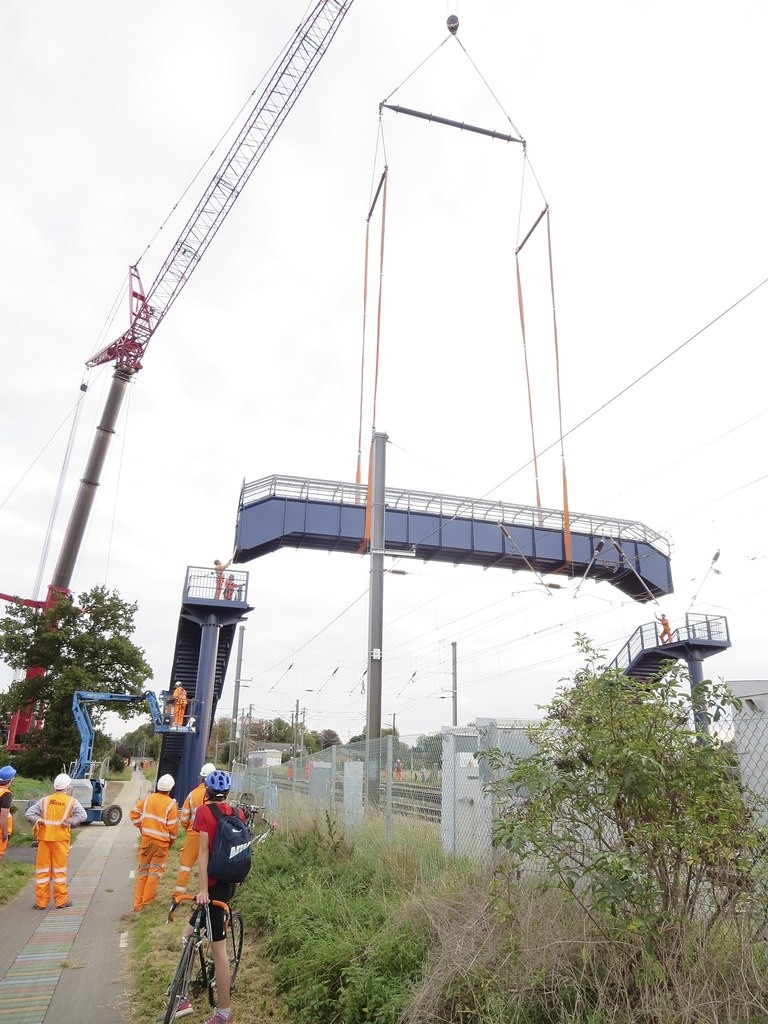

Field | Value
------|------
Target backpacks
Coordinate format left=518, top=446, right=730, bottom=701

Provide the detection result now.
left=206, top=802, right=253, bottom=885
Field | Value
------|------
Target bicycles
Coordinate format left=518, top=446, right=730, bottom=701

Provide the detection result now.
left=162, top=894, right=244, bottom=1024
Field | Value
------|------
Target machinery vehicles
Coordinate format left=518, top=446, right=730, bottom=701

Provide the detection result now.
left=0, top=0, right=570, bottom=771
left=64, top=689, right=203, bottom=826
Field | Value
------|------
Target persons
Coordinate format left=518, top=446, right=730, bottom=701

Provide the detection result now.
left=393, top=759, right=403, bottom=782
left=25, top=773, right=88, bottom=910
left=169, top=681, right=188, bottom=730
left=129, top=773, right=179, bottom=917
left=305, top=757, right=314, bottom=783
left=170, top=762, right=248, bottom=1024
left=214, top=558, right=233, bottom=600
left=287, top=756, right=295, bottom=782
left=143, top=759, right=149, bottom=770
left=222, top=574, right=239, bottom=600
left=419, top=764, right=427, bottom=784
left=0, top=765, right=17, bottom=862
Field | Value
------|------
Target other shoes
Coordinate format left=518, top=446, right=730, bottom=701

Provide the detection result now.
left=31, top=902, right=46, bottom=910
left=170, top=726, right=177, bottom=731
left=59, top=900, right=73, bottom=908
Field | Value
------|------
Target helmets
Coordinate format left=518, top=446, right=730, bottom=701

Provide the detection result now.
left=174, top=681, right=182, bottom=687
left=0, top=765, right=17, bottom=781
left=157, top=774, right=175, bottom=791
left=200, top=763, right=216, bottom=776
left=204, top=770, right=232, bottom=798
left=53, top=773, right=72, bottom=790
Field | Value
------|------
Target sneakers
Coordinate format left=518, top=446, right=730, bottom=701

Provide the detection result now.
left=204, top=1012, right=236, bottom=1024
left=155, top=996, right=193, bottom=1023
